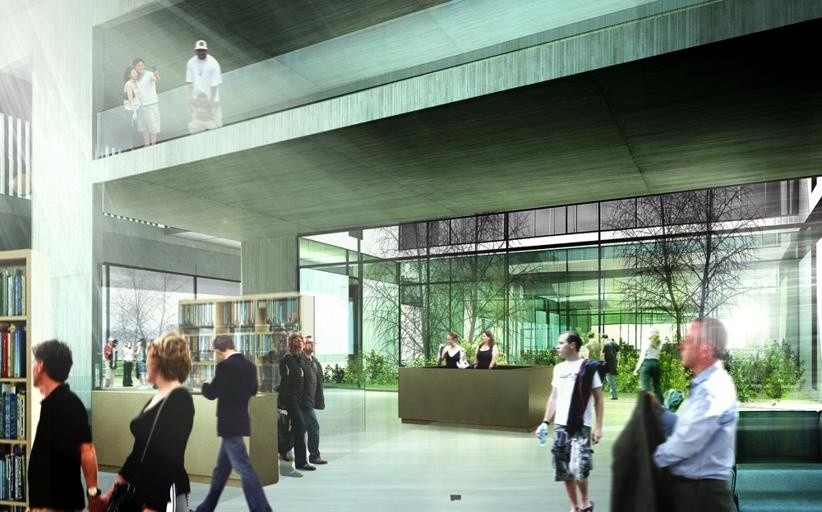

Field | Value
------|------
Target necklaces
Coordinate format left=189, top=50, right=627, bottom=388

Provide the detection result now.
left=196, top=59, right=206, bottom=76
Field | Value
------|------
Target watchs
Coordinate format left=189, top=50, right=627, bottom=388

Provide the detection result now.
left=86, top=486, right=101, bottom=497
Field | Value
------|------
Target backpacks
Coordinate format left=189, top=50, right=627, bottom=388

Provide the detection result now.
left=663, top=389, right=684, bottom=413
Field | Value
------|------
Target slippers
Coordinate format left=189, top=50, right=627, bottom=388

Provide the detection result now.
left=580, top=502, right=594, bottom=512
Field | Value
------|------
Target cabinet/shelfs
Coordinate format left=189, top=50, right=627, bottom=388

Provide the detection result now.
left=177, top=292, right=315, bottom=393
left=0, top=248, right=31, bottom=508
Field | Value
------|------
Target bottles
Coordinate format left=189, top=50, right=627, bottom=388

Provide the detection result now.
left=538, top=425, right=548, bottom=446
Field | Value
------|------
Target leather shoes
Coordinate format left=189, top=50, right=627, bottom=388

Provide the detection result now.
left=280, top=452, right=289, bottom=461
left=309, top=458, right=327, bottom=464
left=296, top=463, right=316, bottom=470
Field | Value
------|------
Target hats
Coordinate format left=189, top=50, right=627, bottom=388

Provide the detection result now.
left=194, top=40, right=208, bottom=50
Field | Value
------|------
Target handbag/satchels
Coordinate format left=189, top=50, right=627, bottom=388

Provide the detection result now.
left=104, top=481, right=146, bottom=512
left=277, top=412, right=295, bottom=454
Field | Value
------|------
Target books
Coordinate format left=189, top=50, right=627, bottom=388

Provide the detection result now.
left=0, top=265, right=28, bottom=512
left=179, top=296, right=299, bottom=392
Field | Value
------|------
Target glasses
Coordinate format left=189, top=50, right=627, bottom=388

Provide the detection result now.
left=305, top=341, right=314, bottom=344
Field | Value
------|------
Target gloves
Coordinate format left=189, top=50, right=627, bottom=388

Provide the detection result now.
left=279, top=410, right=288, bottom=416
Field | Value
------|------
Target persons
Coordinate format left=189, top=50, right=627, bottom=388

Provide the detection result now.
left=475, top=331, right=498, bottom=369
left=195, top=333, right=272, bottom=512
left=537, top=317, right=740, bottom=512
left=115, top=66, right=142, bottom=152
left=276, top=330, right=327, bottom=476
left=133, top=57, right=161, bottom=147
left=27, top=339, right=106, bottom=512
left=188, top=93, right=220, bottom=133
left=437, top=331, right=469, bottom=370
left=185, top=40, right=224, bottom=127
left=95, top=334, right=195, bottom=512
